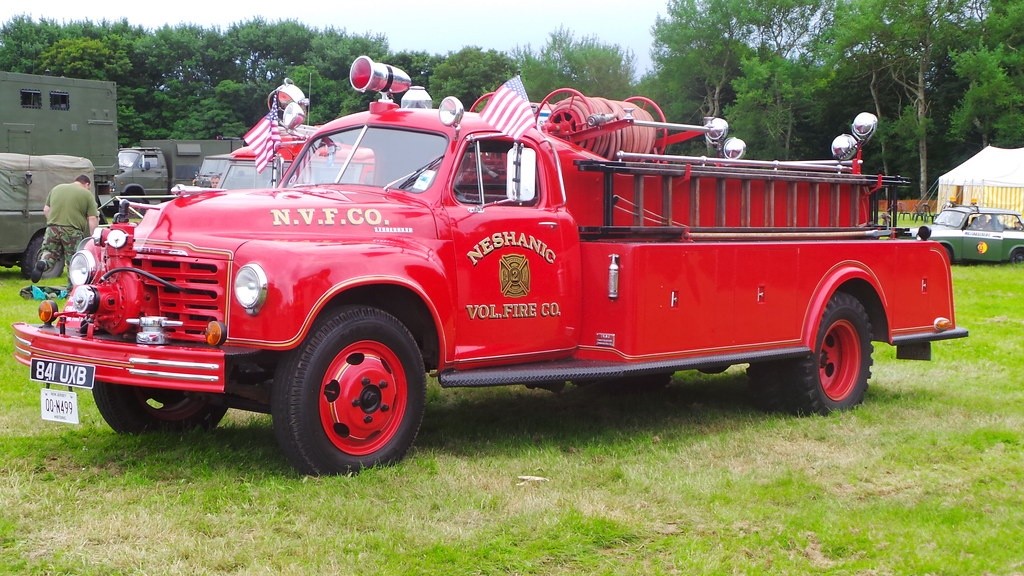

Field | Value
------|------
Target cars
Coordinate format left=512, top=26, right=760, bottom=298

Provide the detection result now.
left=903, top=207, right=1024, bottom=266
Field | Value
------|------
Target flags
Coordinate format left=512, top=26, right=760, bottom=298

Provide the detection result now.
left=244, top=96, right=281, bottom=175
left=480, top=75, right=536, bottom=140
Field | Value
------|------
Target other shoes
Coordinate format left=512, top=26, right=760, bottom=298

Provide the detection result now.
left=31, top=261, right=45, bottom=283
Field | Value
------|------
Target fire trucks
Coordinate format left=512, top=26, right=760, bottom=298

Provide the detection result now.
left=14, top=53, right=970, bottom=474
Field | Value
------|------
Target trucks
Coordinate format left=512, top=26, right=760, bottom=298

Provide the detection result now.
left=0, top=153, right=95, bottom=281
left=108, top=137, right=245, bottom=207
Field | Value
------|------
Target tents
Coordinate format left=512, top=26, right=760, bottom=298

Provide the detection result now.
left=937, top=146, right=1024, bottom=227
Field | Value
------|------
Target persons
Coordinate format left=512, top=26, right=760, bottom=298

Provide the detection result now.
left=973, top=215, right=990, bottom=230
left=210, top=175, right=220, bottom=188
left=31, top=175, right=98, bottom=289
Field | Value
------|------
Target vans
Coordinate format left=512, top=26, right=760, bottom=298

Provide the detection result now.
left=195, top=144, right=375, bottom=194
